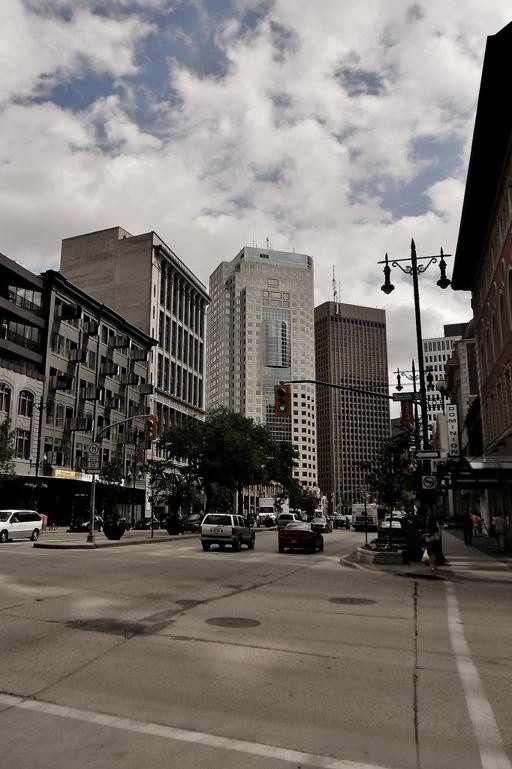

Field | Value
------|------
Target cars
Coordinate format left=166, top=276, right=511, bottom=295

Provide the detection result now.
left=311, top=502, right=406, bottom=536
left=137, top=512, right=203, bottom=534
left=69, top=515, right=104, bottom=532
left=278, top=522, right=325, bottom=552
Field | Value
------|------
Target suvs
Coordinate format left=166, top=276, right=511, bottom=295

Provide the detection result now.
left=200, top=514, right=256, bottom=550
left=276, top=512, right=303, bottom=530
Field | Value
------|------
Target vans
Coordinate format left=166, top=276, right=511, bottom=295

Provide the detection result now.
left=0, top=509, right=44, bottom=545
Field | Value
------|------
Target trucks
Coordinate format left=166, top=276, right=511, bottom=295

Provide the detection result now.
left=256, top=498, right=291, bottom=526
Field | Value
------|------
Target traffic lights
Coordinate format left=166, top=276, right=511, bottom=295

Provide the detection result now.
left=439, top=478, right=448, bottom=488
left=275, top=384, right=291, bottom=417
left=145, top=416, right=157, bottom=440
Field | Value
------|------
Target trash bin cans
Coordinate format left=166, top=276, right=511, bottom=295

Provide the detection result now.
left=41, top=514, right=47, bottom=530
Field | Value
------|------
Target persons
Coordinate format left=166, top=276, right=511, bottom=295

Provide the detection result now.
left=471, top=511, right=482, bottom=537
left=420, top=519, right=443, bottom=572
left=492, top=512, right=506, bottom=550
left=461, top=512, right=473, bottom=545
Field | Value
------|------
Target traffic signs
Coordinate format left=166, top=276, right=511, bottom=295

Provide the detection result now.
left=392, top=393, right=422, bottom=400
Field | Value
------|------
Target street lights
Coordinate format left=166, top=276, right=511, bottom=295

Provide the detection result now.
left=127, top=470, right=142, bottom=529
left=28, top=453, right=49, bottom=510
left=392, top=360, right=436, bottom=548
left=378, top=240, right=456, bottom=564
